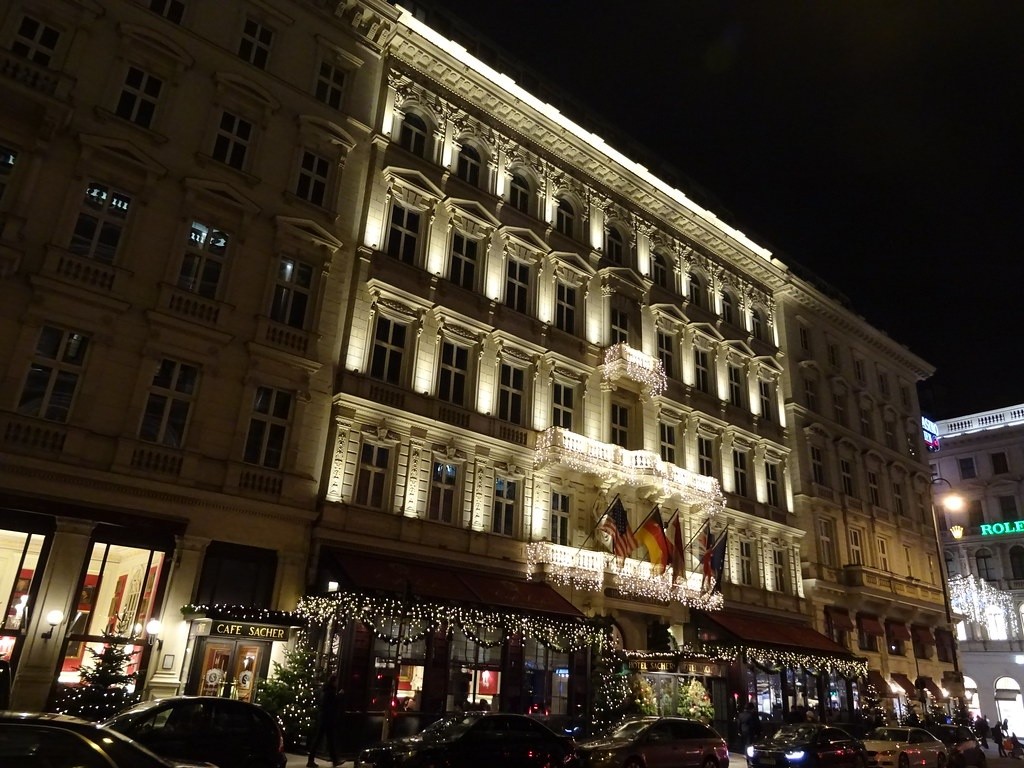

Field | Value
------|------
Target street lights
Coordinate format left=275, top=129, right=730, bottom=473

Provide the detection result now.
left=928, top=475, right=972, bottom=709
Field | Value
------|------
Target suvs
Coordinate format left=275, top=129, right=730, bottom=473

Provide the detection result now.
left=577, top=715, right=730, bottom=768
left=99, top=694, right=290, bottom=768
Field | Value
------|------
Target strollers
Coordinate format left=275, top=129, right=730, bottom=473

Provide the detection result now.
left=1008, top=732, right=1024, bottom=762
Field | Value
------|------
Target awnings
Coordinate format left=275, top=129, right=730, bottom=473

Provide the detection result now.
left=320, top=545, right=587, bottom=621
left=700, top=610, right=853, bottom=658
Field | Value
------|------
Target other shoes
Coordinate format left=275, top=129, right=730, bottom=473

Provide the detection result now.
left=306, top=762, right=319, bottom=768
left=333, top=760, right=346, bottom=767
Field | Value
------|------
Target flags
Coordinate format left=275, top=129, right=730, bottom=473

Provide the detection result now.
left=711, top=531, right=727, bottom=596
left=688, top=523, right=711, bottom=596
left=666, top=511, right=687, bottom=585
left=635, top=508, right=672, bottom=576
left=601, top=498, right=638, bottom=572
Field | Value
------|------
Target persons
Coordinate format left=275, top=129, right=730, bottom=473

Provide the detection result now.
left=788, top=703, right=852, bottom=723
left=737, top=703, right=762, bottom=746
left=306, top=676, right=347, bottom=768
left=976, top=715, right=1024, bottom=760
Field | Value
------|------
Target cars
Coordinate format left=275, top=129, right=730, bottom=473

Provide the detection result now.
left=931, top=724, right=987, bottom=768
left=857, top=726, right=949, bottom=768
left=353, top=710, right=581, bottom=767
left=0, top=710, right=219, bottom=768
left=744, top=722, right=869, bottom=768
